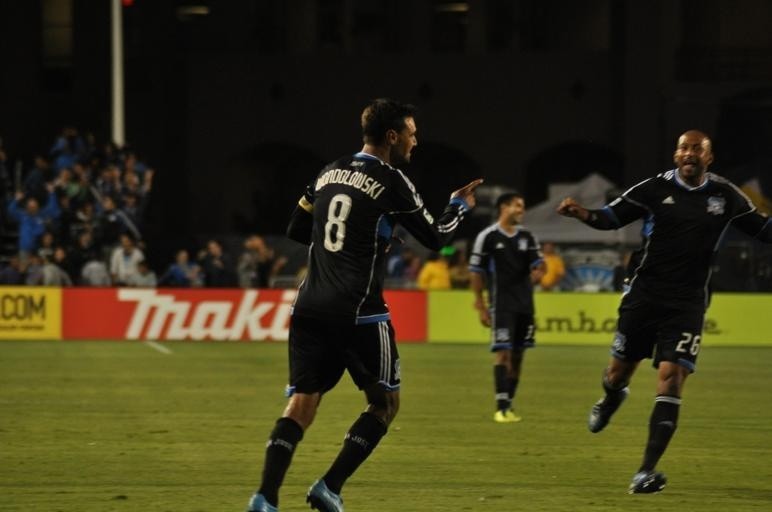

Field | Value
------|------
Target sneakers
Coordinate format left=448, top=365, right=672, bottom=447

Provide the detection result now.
left=306, top=478, right=344, bottom=512
left=493, top=409, right=522, bottom=422
left=588, top=387, right=629, bottom=434
left=628, top=470, right=667, bottom=493
left=247, top=492, right=279, bottom=512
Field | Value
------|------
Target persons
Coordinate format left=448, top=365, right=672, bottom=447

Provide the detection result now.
left=158, top=235, right=286, bottom=287
left=384, top=228, right=566, bottom=291
left=467, top=192, right=548, bottom=423
left=249, top=99, right=485, bottom=511
left=0, top=132, right=156, bottom=288
left=556, top=129, right=772, bottom=483
